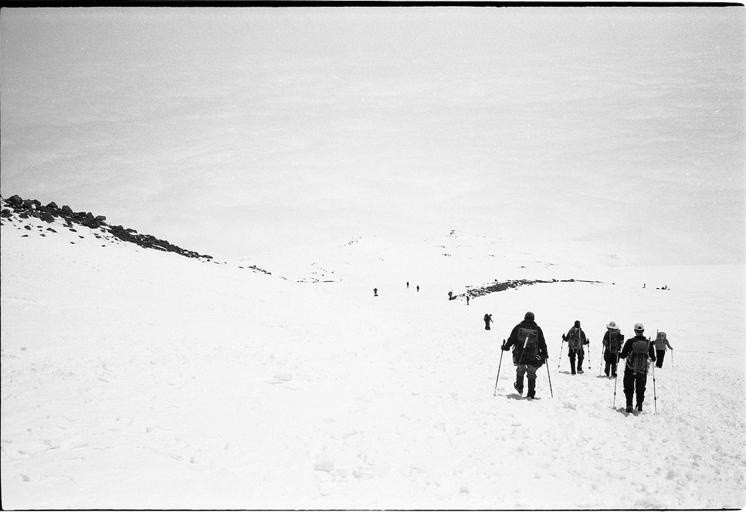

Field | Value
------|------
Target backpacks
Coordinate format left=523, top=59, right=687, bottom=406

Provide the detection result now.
left=569, top=327, right=581, bottom=349
left=657, top=332, right=666, bottom=344
left=609, top=329, right=619, bottom=353
left=517, top=328, right=539, bottom=362
left=627, top=338, right=649, bottom=375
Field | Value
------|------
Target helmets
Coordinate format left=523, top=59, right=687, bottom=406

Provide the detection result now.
left=525, top=312, right=534, bottom=320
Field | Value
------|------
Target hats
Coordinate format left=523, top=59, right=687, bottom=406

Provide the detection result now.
left=634, top=322, right=644, bottom=330
left=575, top=321, right=580, bottom=326
left=606, top=321, right=619, bottom=329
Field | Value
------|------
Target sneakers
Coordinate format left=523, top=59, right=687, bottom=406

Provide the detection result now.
left=626, top=406, right=633, bottom=412
left=577, top=370, right=584, bottom=374
left=514, top=382, right=522, bottom=393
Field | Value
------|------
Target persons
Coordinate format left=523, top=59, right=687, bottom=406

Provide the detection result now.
left=615, top=323, right=657, bottom=414
left=416, top=285, right=419, bottom=293
left=407, top=281, right=409, bottom=288
left=601, top=321, right=624, bottom=377
left=465, top=296, right=470, bottom=305
left=484, top=312, right=494, bottom=330
left=562, top=321, right=589, bottom=376
left=652, top=329, right=674, bottom=370
left=501, top=312, right=550, bottom=398
left=373, top=288, right=378, bottom=296
